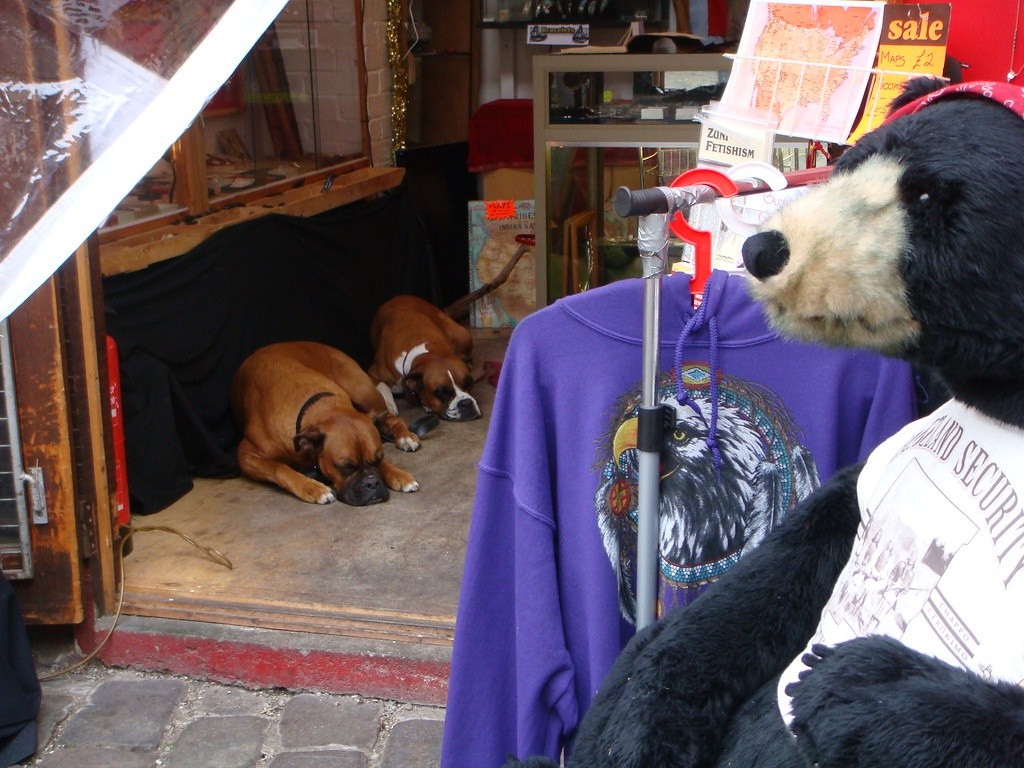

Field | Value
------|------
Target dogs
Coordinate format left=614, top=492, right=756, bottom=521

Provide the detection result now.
left=231, top=293, right=484, bottom=508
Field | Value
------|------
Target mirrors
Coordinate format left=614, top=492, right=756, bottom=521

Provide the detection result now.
left=546, top=146, right=589, bottom=306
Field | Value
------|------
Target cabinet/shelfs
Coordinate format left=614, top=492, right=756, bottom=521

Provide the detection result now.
left=532, top=46, right=738, bottom=315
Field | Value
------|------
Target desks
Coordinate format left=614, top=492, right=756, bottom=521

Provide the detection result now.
left=468, top=98, right=660, bottom=240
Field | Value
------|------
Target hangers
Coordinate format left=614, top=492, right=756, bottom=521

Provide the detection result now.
left=667, top=170, right=738, bottom=295
left=724, top=160, right=789, bottom=277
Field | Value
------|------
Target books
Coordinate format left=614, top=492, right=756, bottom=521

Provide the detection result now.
left=650, top=70, right=720, bottom=90
left=558, top=32, right=704, bottom=54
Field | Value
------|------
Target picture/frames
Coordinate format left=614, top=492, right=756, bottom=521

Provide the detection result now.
left=571, top=211, right=598, bottom=292
